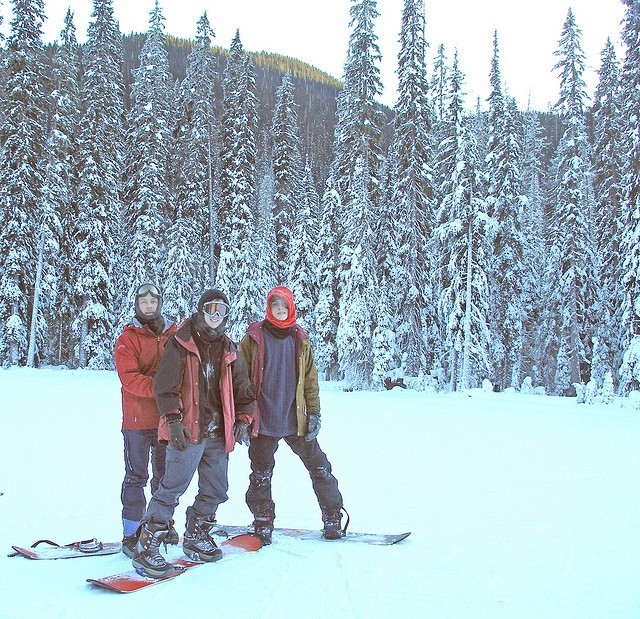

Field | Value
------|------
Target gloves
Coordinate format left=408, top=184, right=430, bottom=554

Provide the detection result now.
left=166, top=413, right=192, bottom=452
left=232, top=420, right=249, bottom=445
left=303, top=412, right=321, bottom=442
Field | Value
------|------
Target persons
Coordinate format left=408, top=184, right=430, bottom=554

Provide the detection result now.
left=239, top=284, right=347, bottom=541
left=114, top=282, right=179, bottom=558
left=132, top=287, right=255, bottom=578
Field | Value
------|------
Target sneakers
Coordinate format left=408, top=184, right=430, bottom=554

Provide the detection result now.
left=253, top=517, right=274, bottom=545
left=164, top=527, right=179, bottom=545
left=122, top=535, right=138, bottom=559
left=323, top=516, right=342, bottom=540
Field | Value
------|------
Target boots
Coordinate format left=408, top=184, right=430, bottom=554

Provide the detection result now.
left=133, top=523, right=174, bottom=579
left=182, top=508, right=223, bottom=563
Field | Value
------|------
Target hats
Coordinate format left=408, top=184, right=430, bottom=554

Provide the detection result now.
left=133, top=283, right=163, bottom=322
left=265, top=285, right=296, bottom=329
left=195, top=289, right=230, bottom=344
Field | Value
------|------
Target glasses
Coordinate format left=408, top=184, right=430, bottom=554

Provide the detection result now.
left=200, top=302, right=231, bottom=317
left=135, top=284, right=161, bottom=297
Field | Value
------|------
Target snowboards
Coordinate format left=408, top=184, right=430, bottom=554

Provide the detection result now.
left=86, top=535, right=262, bottom=593
left=209, top=525, right=411, bottom=546
left=8, top=538, right=123, bottom=560
